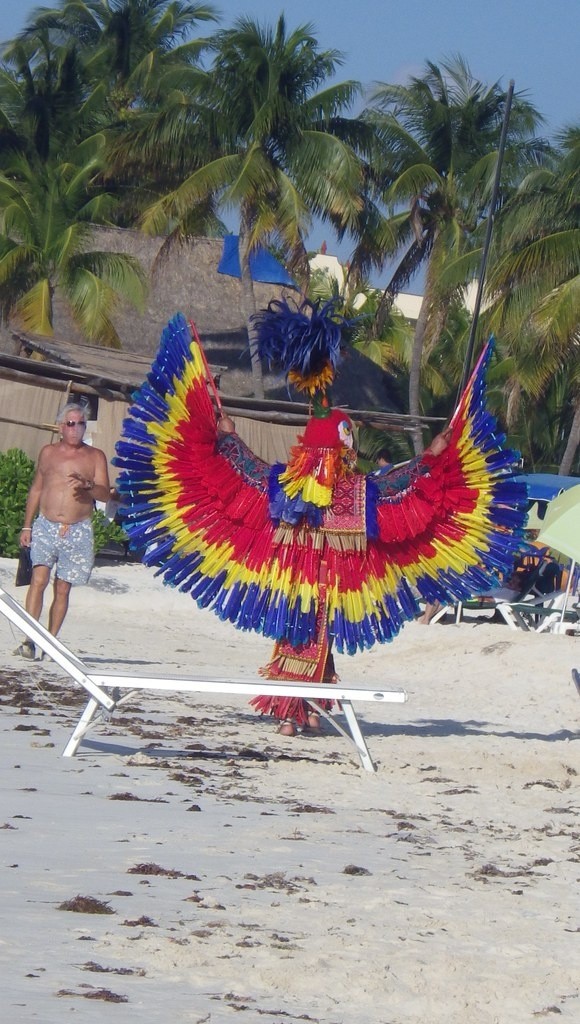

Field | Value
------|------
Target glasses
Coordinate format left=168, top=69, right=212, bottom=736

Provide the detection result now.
left=61, top=421, right=86, bottom=427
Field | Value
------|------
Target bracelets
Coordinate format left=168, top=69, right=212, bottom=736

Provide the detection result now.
left=22, top=527, right=31, bottom=530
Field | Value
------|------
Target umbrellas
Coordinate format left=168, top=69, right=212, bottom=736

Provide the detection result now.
left=501, top=468, right=580, bottom=623
left=536, top=483, right=579, bottom=565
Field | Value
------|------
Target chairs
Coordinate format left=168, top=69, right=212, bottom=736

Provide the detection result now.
left=429, top=576, right=580, bottom=636
left=0, top=585, right=408, bottom=774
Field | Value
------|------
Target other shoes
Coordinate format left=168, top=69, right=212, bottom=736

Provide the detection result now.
left=572, top=669, right=580, bottom=697
left=305, top=716, right=321, bottom=733
left=12, top=640, right=35, bottom=661
left=281, top=721, right=295, bottom=735
left=39, top=649, right=55, bottom=662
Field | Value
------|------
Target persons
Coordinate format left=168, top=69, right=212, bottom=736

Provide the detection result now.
left=14, top=403, right=111, bottom=661
left=215, top=408, right=454, bottom=735
left=373, top=447, right=393, bottom=475
left=419, top=570, right=526, bottom=625
